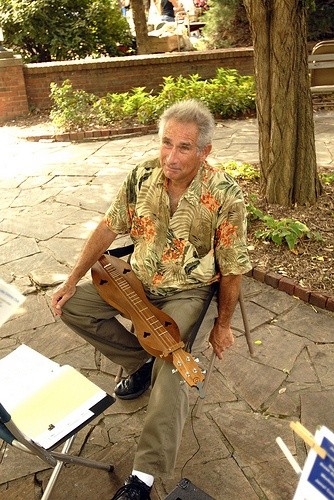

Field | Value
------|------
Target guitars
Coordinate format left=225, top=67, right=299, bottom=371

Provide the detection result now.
left=91, top=254, right=207, bottom=399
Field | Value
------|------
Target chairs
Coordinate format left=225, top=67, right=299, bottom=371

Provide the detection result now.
left=310, top=40, right=334, bottom=107
left=0, top=344, right=114, bottom=500
left=103, top=244, right=256, bottom=384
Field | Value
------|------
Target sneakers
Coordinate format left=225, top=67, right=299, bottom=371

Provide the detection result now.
left=114, top=357, right=156, bottom=399
left=111, top=474, right=154, bottom=500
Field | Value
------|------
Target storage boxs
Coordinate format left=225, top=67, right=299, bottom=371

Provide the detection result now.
left=149, top=34, right=181, bottom=54
